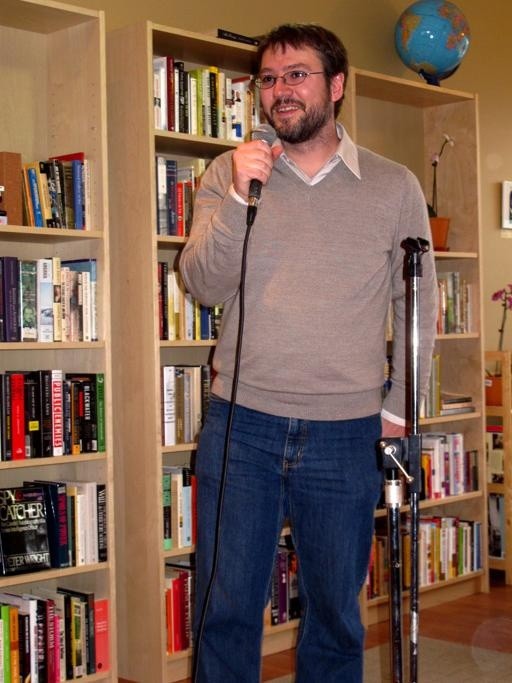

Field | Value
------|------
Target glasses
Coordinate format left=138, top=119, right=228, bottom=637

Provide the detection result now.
left=250, top=70, right=326, bottom=89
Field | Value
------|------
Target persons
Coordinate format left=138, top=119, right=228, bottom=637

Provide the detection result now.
left=176, top=21, right=439, bottom=682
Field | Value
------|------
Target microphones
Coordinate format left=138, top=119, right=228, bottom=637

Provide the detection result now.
left=247, top=122, right=276, bottom=227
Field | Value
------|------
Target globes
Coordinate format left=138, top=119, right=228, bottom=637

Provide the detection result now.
left=394, top=0, right=471, bottom=88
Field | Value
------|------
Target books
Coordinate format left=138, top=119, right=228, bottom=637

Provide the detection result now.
left=1, top=256, right=100, bottom=345
left=270, top=536, right=303, bottom=625
left=156, top=156, right=226, bottom=341
left=203, top=27, right=261, bottom=47
left=2, top=150, right=91, bottom=230
left=151, top=56, right=271, bottom=143
left=162, top=364, right=217, bottom=655
left=366, top=431, right=482, bottom=600
left=384, top=271, right=476, bottom=419
left=1, top=370, right=109, bottom=683
